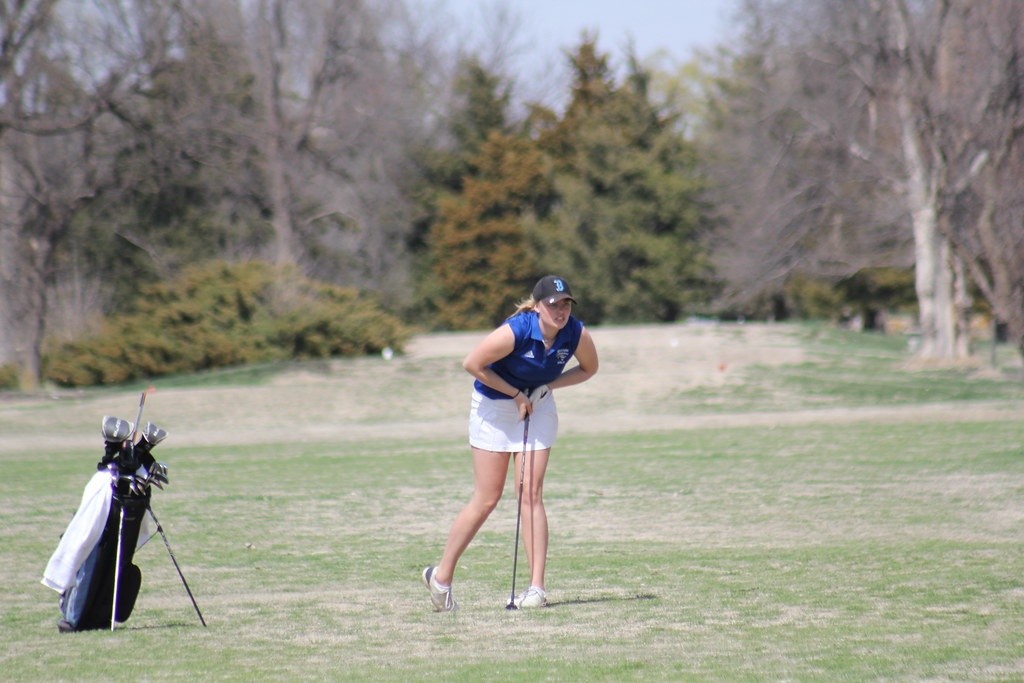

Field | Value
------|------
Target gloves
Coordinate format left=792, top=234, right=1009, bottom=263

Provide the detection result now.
left=528, top=385, right=552, bottom=411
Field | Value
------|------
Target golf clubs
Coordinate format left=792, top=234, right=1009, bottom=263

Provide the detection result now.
left=103, top=393, right=169, bottom=498
left=507, top=387, right=530, bottom=609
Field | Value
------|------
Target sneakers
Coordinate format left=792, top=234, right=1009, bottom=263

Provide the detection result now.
left=507, top=585, right=548, bottom=608
left=422, top=567, right=460, bottom=613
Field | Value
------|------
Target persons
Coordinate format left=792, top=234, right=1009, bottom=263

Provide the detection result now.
left=423, top=276, right=598, bottom=610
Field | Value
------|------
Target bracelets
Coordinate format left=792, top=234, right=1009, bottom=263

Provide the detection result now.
left=513, top=390, right=521, bottom=399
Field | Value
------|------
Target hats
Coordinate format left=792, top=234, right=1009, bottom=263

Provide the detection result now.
left=532, top=275, right=578, bottom=306
